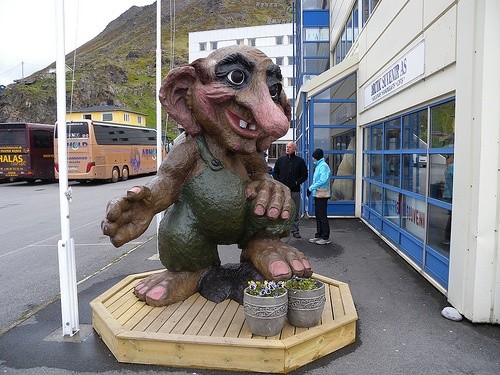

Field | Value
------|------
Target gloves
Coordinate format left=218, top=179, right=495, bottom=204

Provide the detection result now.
left=307, top=189, right=311, bottom=198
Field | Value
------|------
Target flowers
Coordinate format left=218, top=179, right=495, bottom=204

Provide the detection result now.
left=286, top=275, right=318, bottom=290
left=246, top=280, right=286, bottom=297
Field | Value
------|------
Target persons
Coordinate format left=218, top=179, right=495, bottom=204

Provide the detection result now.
left=306, top=148, right=332, bottom=244
left=442, top=156, right=453, bottom=245
left=274, top=141, right=308, bottom=238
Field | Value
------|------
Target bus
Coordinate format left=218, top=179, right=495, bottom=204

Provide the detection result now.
left=53, top=120, right=173, bottom=183
left=1, top=123, right=55, bottom=184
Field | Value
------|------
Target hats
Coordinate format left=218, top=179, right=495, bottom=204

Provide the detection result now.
left=312, top=148, right=324, bottom=161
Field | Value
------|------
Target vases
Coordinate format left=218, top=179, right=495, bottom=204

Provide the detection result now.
left=287, top=278, right=325, bottom=328
left=243, top=287, right=288, bottom=337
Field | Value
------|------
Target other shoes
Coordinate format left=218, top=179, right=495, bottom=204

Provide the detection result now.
left=293, top=233, right=301, bottom=238
left=442, top=238, right=450, bottom=244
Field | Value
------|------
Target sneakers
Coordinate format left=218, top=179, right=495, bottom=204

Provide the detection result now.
left=316, top=238, right=331, bottom=244
left=308, top=237, right=321, bottom=243
